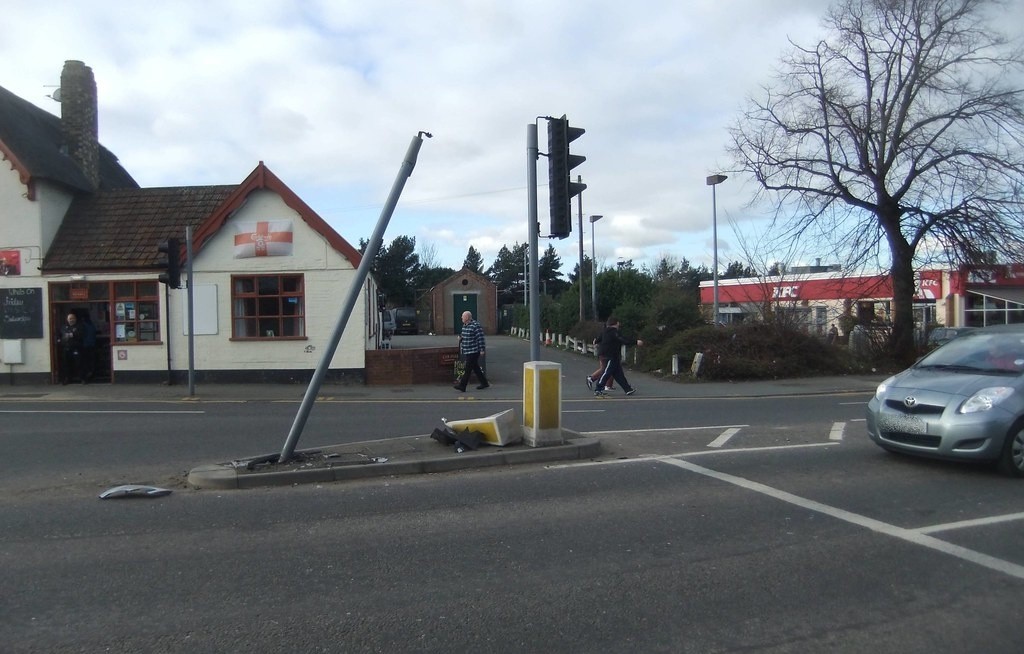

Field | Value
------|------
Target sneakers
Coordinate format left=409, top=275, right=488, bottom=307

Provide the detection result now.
left=626, top=388, right=636, bottom=395
left=585, top=376, right=594, bottom=390
left=594, top=390, right=604, bottom=395
left=604, top=385, right=616, bottom=391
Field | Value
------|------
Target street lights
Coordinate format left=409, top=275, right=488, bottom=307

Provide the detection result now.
left=706, top=175, right=728, bottom=329
left=590, top=214, right=603, bottom=322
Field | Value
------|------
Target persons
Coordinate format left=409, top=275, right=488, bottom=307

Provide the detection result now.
left=825, top=324, right=838, bottom=344
left=57, top=312, right=84, bottom=387
left=585, top=316, right=637, bottom=397
left=81, top=312, right=97, bottom=359
left=452, top=310, right=490, bottom=392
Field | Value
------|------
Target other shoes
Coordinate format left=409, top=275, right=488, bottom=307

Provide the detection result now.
left=476, top=383, right=490, bottom=389
left=453, top=384, right=467, bottom=392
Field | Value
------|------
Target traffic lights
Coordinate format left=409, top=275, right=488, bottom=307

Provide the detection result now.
left=158, top=237, right=181, bottom=289
left=546, top=115, right=588, bottom=240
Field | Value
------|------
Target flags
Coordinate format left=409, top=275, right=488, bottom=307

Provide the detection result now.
left=233, top=220, right=293, bottom=260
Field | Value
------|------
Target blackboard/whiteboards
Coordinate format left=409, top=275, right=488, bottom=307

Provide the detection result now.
left=181, top=283, right=218, bottom=335
left=0, top=288, right=44, bottom=339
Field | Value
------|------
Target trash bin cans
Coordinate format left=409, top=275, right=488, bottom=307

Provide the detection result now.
left=445, top=408, right=521, bottom=447
left=521, top=361, right=565, bottom=447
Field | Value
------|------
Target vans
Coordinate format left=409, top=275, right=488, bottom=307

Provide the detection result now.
left=393, top=308, right=419, bottom=336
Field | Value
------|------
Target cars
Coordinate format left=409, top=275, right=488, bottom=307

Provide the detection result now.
left=383, top=311, right=396, bottom=337
left=868, top=325, right=1024, bottom=479
